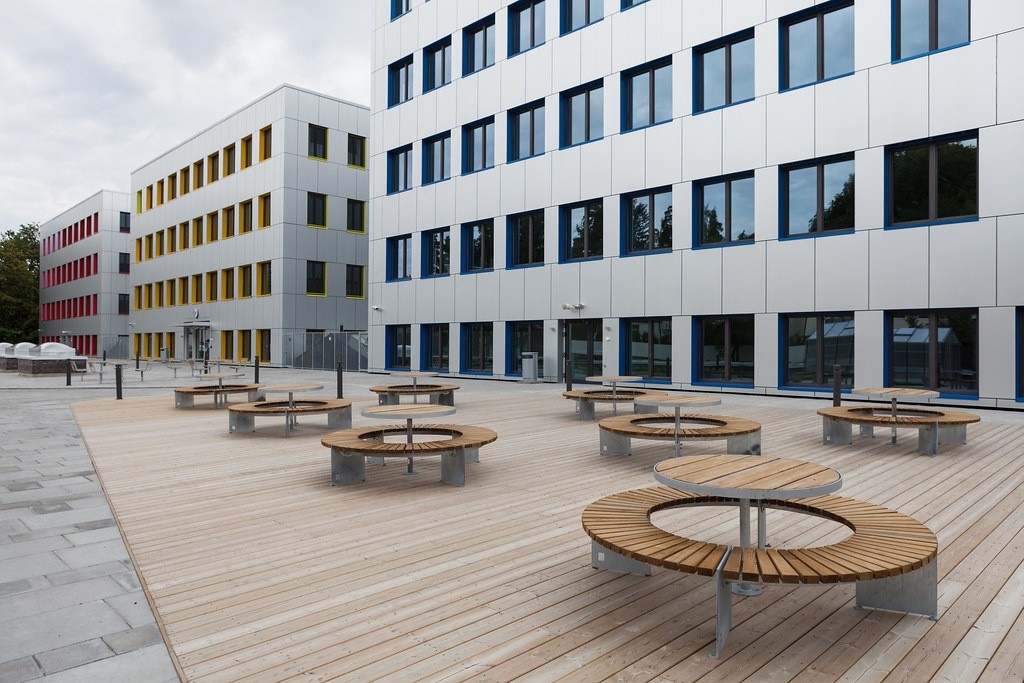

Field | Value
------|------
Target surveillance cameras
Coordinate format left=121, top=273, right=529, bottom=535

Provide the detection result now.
left=371, top=305, right=378, bottom=310
left=128, top=322, right=131, bottom=325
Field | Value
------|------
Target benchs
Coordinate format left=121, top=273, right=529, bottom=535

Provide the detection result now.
left=174, top=384, right=267, bottom=407
left=814, top=405, right=980, bottom=456
left=564, top=387, right=670, bottom=413
left=227, top=397, right=352, bottom=429
left=581, top=488, right=940, bottom=662
left=322, top=421, right=499, bottom=488
left=593, top=413, right=763, bottom=455
left=369, top=385, right=461, bottom=406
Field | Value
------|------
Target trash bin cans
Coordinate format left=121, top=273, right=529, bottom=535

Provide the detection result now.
left=160, top=347, right=168, bottom=363
left=521, top=352, right=539, bottom=380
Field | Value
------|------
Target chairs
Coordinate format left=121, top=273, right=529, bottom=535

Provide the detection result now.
left=70, top=358, right=248, bottom=382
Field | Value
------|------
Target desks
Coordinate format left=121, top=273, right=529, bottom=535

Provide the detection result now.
left=587, top=376, right=642, bottom=415
left=653, top=453, right=843, bottom=596
left=256, top=385, right=324, bottom=425
left=850, top=386, right=941, bottom=444
left=632, top=395, right=723, bottom=458
left=193, top=372, right=243, bottom=406
left=391, top=373, right=440, bottom=404
left=359, top=403, right=457, bottom=477
